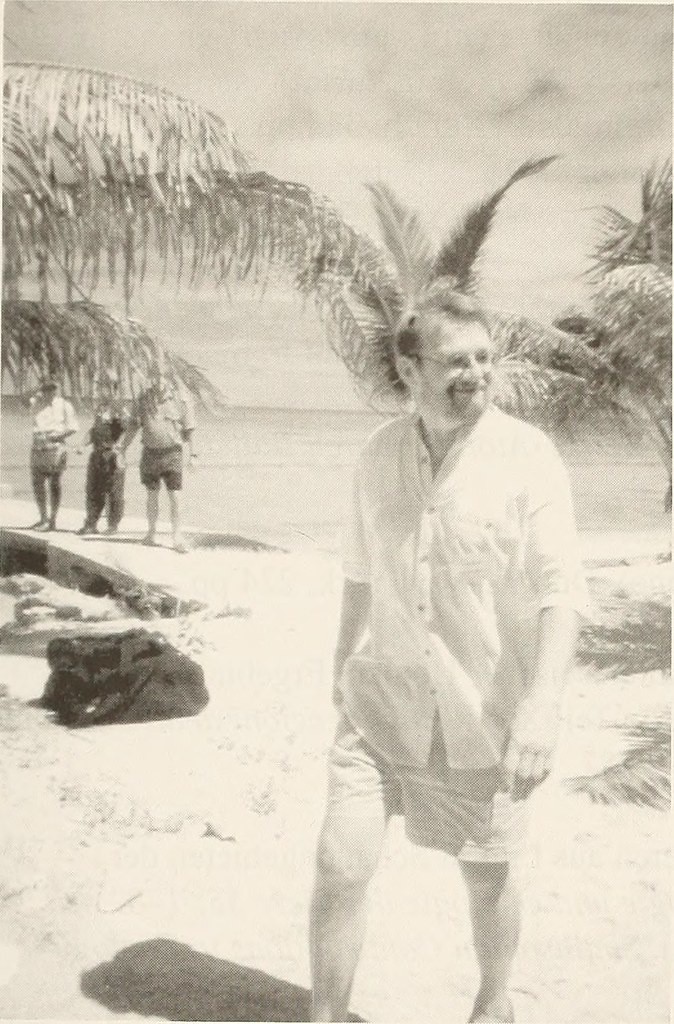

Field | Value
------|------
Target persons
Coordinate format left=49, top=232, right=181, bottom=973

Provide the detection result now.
left=76, top=370, right=140, bottom=536
left=123, top=358, right=196, bottom=552
left=28, top=373, right=76, bottom=531
left=308, top=291, right=574, bottom=1024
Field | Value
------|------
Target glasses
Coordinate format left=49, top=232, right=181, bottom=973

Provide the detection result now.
left=412, top=350, right=499, bottom=372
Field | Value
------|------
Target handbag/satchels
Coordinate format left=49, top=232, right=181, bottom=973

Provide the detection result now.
left=43, top=630, right=209, bottom=726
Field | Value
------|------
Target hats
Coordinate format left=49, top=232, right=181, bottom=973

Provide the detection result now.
left=41, top=375, right=60, bottom=390
left=91, top=369, right=125, bottom=386
